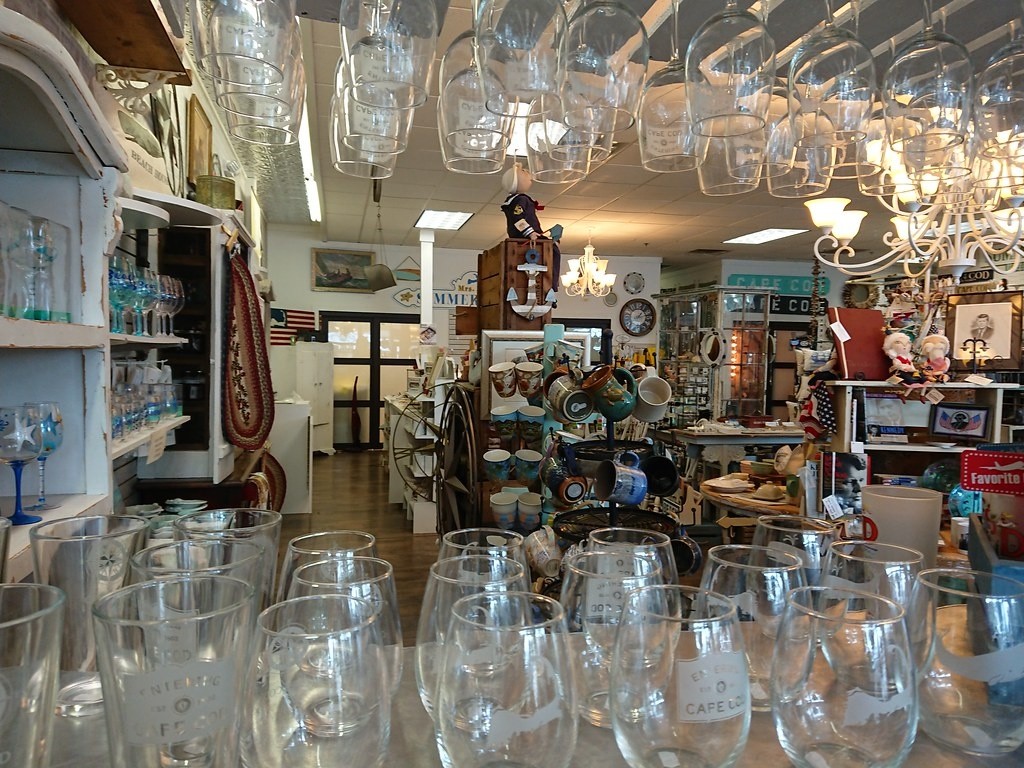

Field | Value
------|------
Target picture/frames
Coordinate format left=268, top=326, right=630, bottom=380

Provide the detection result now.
left=185, top=93, right=213, bottom=189
left=311, top=247, right=377, bottom=295
left=927, top=401, right=994, bottom=443
left=945, top=290, right=1024, bottom=373
left=480, top=329, right=591, bottom=421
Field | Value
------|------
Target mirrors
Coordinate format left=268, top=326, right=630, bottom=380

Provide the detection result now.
left=551, top=316, right=613, bottom=478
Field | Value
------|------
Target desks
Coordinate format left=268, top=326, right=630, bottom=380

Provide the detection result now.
left=383, top=394, right=438, bottom=534
left=141, top=440, right=288, bottom=529
left=0, top=600, right=989, bottom=768
left=668, top=427, right=806, bottom=537
left=699, top=475, right=801, bottom=518
left=824, top=380, right=1020, bottom=453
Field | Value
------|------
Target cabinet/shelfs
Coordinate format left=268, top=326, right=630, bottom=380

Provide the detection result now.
left=109, top=331, right=191, bottom=461
left=0, top=5, right=129, bottom=586
left=651, top=284, right=779, bottom=425
left=267, top=399, right=314, bottom=515
left=270, top=341, right=336, bottom=455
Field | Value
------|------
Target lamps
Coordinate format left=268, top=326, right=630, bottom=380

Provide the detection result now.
left=806, top=106, right=1024, bottom=286
left=560, top=225, right=617, bottom=298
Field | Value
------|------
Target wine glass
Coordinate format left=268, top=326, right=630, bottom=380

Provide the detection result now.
left=0, top=406, right=43, bottom=525
left=22, top=400, right=64, bottom=511
left=109, top=256, right=185, bottom=339
left=190, top=0, right=1024, bottom=215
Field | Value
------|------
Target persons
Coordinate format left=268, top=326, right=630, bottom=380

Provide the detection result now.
left=884, top=333, right=930, bottom=405
left=501, top=162, right=561, bottom=309
left=952, top=414, right=968, bottom=429
left=837, top=453, right=866, bottom=507
left=992, top=279, right=1007, bottom=291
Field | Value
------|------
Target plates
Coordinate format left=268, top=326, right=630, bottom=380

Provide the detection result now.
left=948, top=483, right=982, bottom=517
left=704, top=479, right=755, bottom=492
left=125, top=497, right=208, bottom=538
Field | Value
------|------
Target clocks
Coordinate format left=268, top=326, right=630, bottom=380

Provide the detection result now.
left=619, top=298, right=656, bottom=337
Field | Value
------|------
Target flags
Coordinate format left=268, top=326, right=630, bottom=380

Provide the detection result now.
left=939, top=412, right=952, bottom=429
left=270, top=308, right=315, bottom=345
left=965, top=414, right=981, bottom=430
left=798, top=381, right=837, bottom=439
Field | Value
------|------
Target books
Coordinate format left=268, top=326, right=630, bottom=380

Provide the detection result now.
left=819, top=451, right=867, bottom=514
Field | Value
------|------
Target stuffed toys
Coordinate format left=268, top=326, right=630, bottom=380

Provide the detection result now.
left=922, top=335, right=951, bottom=382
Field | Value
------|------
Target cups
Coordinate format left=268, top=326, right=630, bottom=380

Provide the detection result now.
left=483, top=339, right=680, bottom=503
left=111, top=383, right=183, bottom=439
left=174, top=508, right=282, bottom=691
left=238, top=507, right=1024, bottom=768
left=92, top=576, right=254, bottom=768
left=515, top=492, right=545, bottom=531
left=864, top=487, right=943, bottom=645
left=0, top=516, right=145, bottom=768
left=129, top=538, right=262, bottom=750
left=950, top=517, right=969, bottom=547
left=491, top=492, right=517, bottom=530
left=956, top=522, right=970, bottom=555
left=785, top=474, right=802, bottom=504
left=0, top=201, right=72, bottom=323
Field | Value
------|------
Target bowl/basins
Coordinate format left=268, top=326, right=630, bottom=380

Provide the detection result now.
left=730, top=459, right=774, bottom=479
